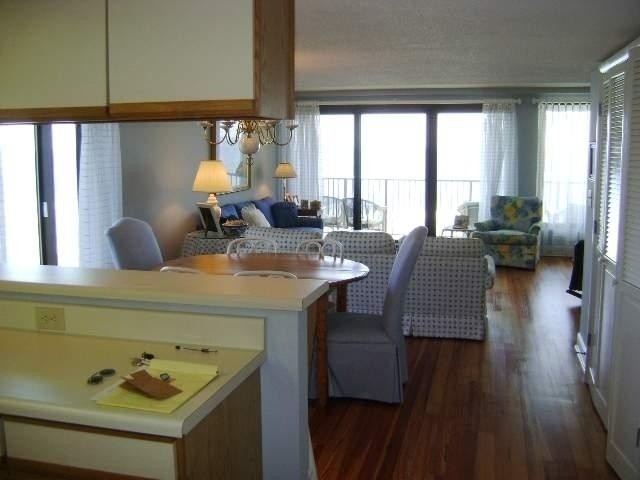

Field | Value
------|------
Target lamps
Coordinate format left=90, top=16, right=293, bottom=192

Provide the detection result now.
left=192, top=160, right=234, bottom=205
left=272, top=162, right=297, bottom=202
left=201, top=117, right=299, bottom=156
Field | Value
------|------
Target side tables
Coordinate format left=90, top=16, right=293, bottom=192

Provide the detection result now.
left=183, top=230, right=240, bottom=255
left=440, top=225, right=477, bottom=238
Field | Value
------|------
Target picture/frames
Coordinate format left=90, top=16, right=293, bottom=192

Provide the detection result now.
left=198, top=206, right=223, bottom=238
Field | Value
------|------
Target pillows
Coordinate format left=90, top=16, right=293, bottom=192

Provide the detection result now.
left=271, top=202, right=298, bottom=228
left=241, top=207, right=270, bottom=227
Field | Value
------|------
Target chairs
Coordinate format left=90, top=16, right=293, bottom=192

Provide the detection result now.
left=105, top=216, right=165, bottom=271
left=321, top=195, right=348, bottom=229
left=344, top=198, right=389, bottom=232
left=310, top=226, right=429, bottom=407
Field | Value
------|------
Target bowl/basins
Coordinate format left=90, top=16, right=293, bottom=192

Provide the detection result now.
left=222, top=223, right=249, bottom=237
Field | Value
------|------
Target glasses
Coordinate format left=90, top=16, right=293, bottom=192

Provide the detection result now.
left=86, top=367, right=115, bottom=384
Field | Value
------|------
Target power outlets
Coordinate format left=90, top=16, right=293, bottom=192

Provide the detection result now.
left=35, top=306, right=66, bottom=330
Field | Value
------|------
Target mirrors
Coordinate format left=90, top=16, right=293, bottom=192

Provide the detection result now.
left=211, top=120, right=252, bottom=196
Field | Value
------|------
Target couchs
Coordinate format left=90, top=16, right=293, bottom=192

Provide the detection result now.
left=472, top=195, right=543, bottom=271
left=221, top=197, right=324, bottom=236
left=245, top=229, right=395, bottom=317
left=396, top=237, right=496, bottom=342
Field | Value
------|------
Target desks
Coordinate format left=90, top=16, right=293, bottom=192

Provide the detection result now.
left=0, top=324, right=268, bottom=480
left=151, top=250, right=371, bottom=407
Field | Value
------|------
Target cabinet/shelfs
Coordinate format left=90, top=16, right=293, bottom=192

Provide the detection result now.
left=575, top=40, right=640, bottom=480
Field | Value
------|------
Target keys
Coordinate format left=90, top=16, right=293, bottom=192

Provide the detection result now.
left=129, top=352, right=154, bottom=367
